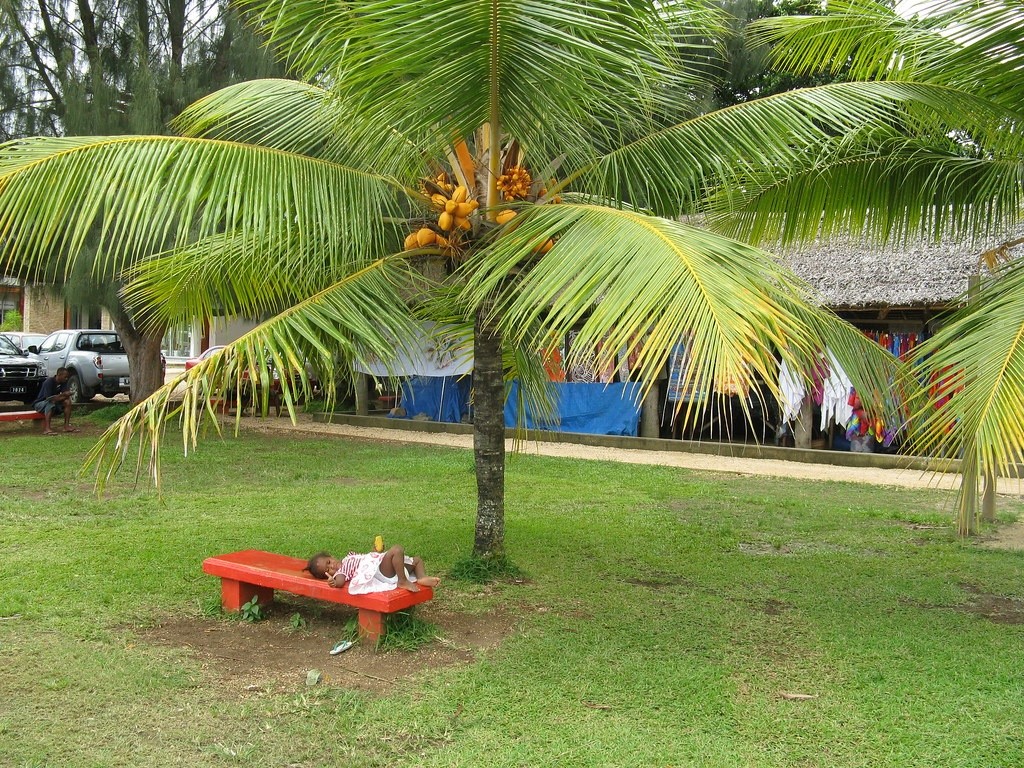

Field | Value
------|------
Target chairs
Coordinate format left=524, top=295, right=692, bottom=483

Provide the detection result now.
left=93, top=338, right=105, bottom=348
left=80, top=339, right=92, bottom=349
left=107, top=336, right=123, bottom=351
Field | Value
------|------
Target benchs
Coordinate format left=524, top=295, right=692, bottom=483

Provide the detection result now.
left=0, top=410, right=51, bottom=431
left=211, top=395, right=282, bottom=414
left=203, top=549, right=434, bottom=647
left=378, top=396, right=401, bottom=409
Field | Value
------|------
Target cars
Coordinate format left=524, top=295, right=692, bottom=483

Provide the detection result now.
left=182, top=344, right=317, bottom=397
left=1, top=332, right=48, bottom=353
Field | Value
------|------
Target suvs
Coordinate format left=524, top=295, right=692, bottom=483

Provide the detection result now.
left=0, top=334, right=48, bottom=405
left=25, top=328, right=167, bottom=403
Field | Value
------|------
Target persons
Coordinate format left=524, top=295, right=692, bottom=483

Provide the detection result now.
left=303, top=543, right=441, bottom=595
left=32, top=367, right=77, bottom=436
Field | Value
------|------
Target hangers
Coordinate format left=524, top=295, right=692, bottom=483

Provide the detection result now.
left=858, top=328, right=918, bottom=338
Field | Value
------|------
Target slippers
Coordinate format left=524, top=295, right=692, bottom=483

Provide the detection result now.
left=330, top=641, right=352, bottom=655
left=42, top=431, right=58, bottom=436
left=63, top=428, right=80, bottom=432
left=306, top=669, right=322, bottom=686
